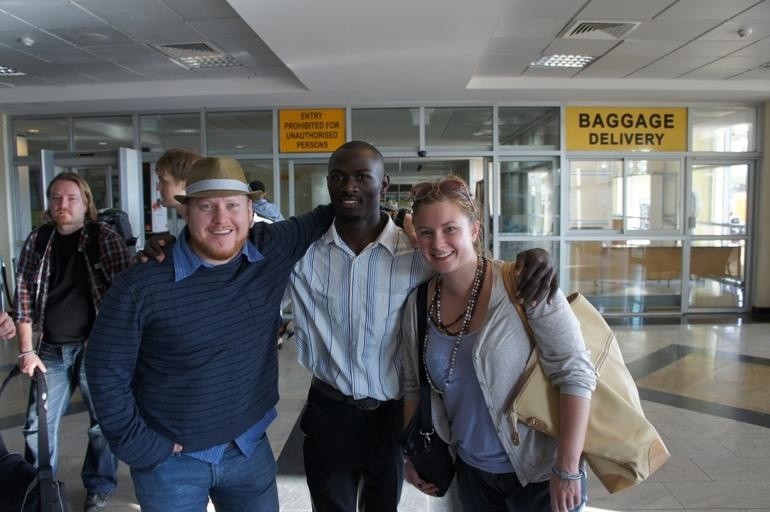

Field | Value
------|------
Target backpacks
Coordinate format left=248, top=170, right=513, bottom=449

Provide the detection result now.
left=32, top=208, right=138, bottom=288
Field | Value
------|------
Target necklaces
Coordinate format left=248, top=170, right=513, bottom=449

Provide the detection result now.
left=420, top=254, right=487, bottom=398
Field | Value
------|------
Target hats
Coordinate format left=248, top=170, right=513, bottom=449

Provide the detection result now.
left=173, top=156, right=265, bottom=205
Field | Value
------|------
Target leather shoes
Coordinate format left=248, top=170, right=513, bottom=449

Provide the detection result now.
left=81, top=486, right=117, bottom=512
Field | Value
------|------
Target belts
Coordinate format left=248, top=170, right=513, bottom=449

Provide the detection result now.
left=311, top=375, right=400, bottom=412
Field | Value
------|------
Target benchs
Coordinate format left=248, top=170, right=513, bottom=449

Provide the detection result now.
left=570, top=240, right=742, bottom=294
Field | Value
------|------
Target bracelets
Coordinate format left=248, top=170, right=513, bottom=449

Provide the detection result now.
left=400, top=446, right=413, bottom=465
left=15, top=349, right=37, bottom=358
left=550, top=464, right=584, bottom=484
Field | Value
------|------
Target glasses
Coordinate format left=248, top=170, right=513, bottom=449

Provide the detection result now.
left=410, top=180, right=475, bottom=215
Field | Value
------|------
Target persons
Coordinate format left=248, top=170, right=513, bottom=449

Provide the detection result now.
left=155, top=144, right=204, bottom=224
left=10, top=170, right=134, bottom=512
left=0, top=308, right=19, bottom=343
left=79, top=154, right=420, bottom=512
left=134, top=138, right=560, bottom=512
left=248, top=212, right=295, bottom=350
left=396, top=171, right=603, bottom=512
left=247, top=178, right=287, bottom=224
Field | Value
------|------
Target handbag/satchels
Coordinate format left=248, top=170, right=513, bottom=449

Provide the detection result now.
left=500, top=260, right=671, bottom=495
left=0, top=452, right=67, bottom=512
left=400, top=380, right=456, bottom=497
